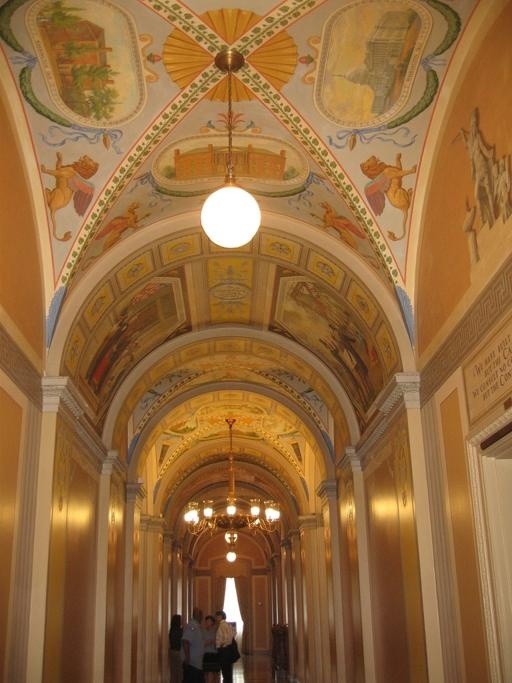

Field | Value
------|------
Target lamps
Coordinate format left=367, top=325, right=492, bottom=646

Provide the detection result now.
left=199, top=47, right=262, bottom=249
left=181, top=415, right=282, bottom=533
left=224, top=532, right=239, bottom=562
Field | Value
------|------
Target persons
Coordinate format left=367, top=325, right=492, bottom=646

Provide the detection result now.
left=84, top=307, right=141, bottom=397
left=169, top=607, right=241, bottom=683
left=451, top=105, right=512, bottom=227
left=318, top=323, right=376, bottom=414
